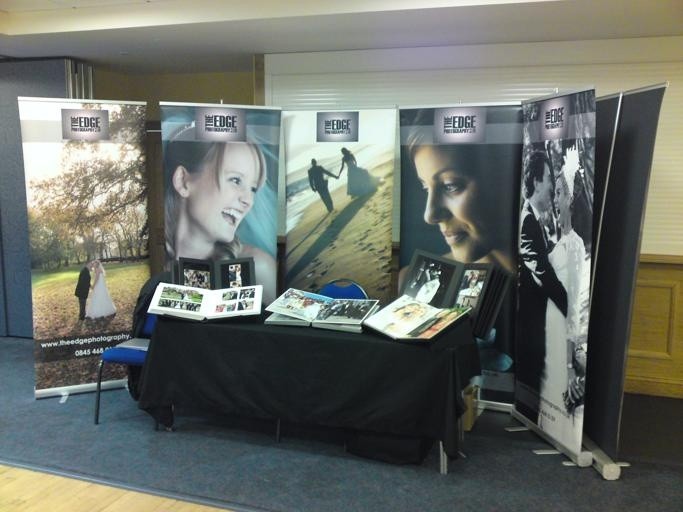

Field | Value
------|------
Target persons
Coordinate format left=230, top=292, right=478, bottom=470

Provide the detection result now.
left=85, top=261, right=117, bottom=319
left=339, top=148, right=386, bottom=199
left=74, top=260, right=92, bottom=320
left=397, top=124, right=516, bottom=301
left=163, top=124, right=278, bottom=306
left=308, top=159, right=339, bottom=212
left=516, top=150, right=589, bottom=417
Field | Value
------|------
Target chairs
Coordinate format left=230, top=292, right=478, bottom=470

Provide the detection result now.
left=92, top=271, right=169, bottom=430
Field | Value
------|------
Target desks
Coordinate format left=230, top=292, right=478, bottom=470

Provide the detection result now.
left=152, top=304, right=471, bottom=476
